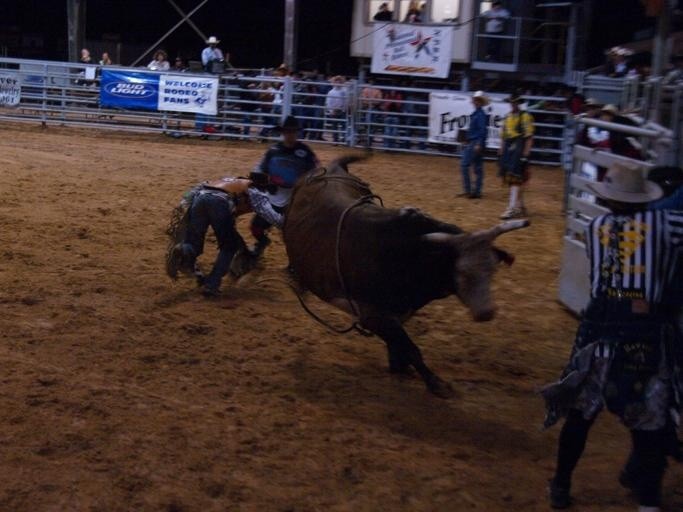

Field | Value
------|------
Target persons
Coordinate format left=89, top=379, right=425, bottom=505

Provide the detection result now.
left=98, top=53, right=111, bottom=77
left=173, top=65, right=456, bottom=154
left=175, top=56, right=190, bottom=72
left=401, top=2, right=422, bottom=24
left=539, top=160, right=682, bottom=510
left=201, top=37, right=224, bottom=73
left=562, top=93, right=643, bottom=202
left=470, top=77, right=573, bottom=162
left=373, top=3, right=394, bottom=21
left=497, top=93, right=534, bottom=219
left=147, top=51, right=170, bottom=71
left=171, top=173, right=286, bottom=295
left=79, top=49, right=98, bottom=89
left=457, top=91, right=490, bottom=200
left=586, top=46, right=682, bottom=129
left=250, top=115, right=319, bottom=271
left=480, top=2, right=511, bottom=63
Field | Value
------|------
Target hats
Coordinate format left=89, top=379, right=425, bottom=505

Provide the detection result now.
left=472, top=91, right=489, bottom=107
left=582, top=159, right=664, bottom=205
left=600, top=103, right=617, bottom=116
left=204, top=36, right=221, bottom=44
left=503, top=93, right=524, bottom=105
left=279, top=63, right=288, bottom=71
left=154, top=49, right=168, bottom=60
left=273, top=116, right=303, bottom=133
left=581, top=97, right=604, bottom=107
left=329, top=74, right=345, bottom=82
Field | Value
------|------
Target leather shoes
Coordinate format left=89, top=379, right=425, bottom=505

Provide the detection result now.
left=468, top=192, right=480, bottom=198
left=457, top=191, right=470, bottom=197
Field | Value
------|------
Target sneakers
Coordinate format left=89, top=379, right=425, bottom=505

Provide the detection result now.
left=199, top=283, right=222, bottom=296
left=250, top=236, right=271, bottom=259
left=500, top=206, right=520, bottom=219
left=548, top=476, right=572, bottom=509
left=183, top=242, right=196, bottom=275
left=515, top=207, right=528, bottom=216
left=615, top=469, right=661, bottom=511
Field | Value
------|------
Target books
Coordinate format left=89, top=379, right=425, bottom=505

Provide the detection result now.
left=457, top=130, right=470, bottom=143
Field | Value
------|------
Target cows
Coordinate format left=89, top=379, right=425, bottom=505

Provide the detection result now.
left=281, top=147, right=532, bottom=401
